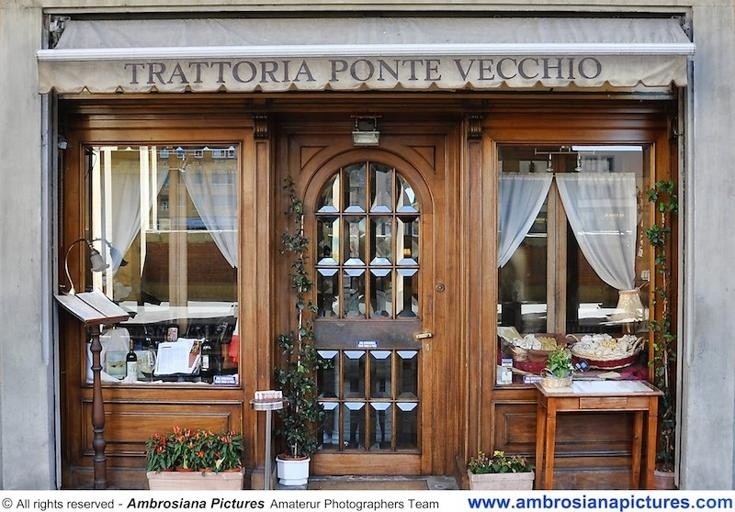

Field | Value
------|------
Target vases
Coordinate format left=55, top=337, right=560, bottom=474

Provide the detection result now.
left=466, top=467, right=536, bottom=490
left=145, top=464, right=246, bottom=490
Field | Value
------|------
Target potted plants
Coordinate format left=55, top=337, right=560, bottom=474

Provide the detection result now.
left=271, top=172, right=338, bottom=487
left=644, top=163, right=679, bottom=491
left=540, top=345, right=577, bottom=391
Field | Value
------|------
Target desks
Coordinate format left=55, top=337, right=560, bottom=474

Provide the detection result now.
left=532, top=379, right=666, bottom=490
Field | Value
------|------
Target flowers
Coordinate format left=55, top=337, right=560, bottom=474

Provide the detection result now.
left=466, top=448, right=538, bottom=474
left=142, top=424, right=247, bottom=476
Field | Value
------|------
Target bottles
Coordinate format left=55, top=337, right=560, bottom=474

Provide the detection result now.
left=125, top=337, right=138, bottom=381
left=201, top=325, right=217, bottom=384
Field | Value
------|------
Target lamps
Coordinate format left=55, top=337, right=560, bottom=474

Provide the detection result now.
left=350, top=114, right=383, bottom=148
left=60, top=237, right=110, bottom=300
